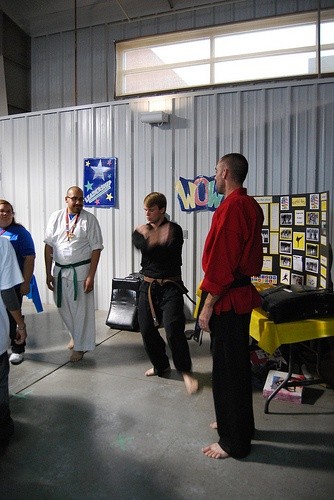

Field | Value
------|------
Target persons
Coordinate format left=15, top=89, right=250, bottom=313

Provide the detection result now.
left=132, top=192, right=199, bottom=394
left=198, top=153, right=264, bottom=460
left=0, top=200, right=43, bottom=363
left=0, top=236, right=27, bottom=439
left=44, top=186, right=104, bottom=362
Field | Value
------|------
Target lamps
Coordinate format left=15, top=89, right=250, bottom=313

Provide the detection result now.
left=139, top=111, right=168, bottom=127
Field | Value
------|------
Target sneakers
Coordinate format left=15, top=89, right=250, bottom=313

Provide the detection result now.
left=9, top=352, right=24, bottom=362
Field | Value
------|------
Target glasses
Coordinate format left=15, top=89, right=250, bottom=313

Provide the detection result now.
left=66, top=196, right=84, bottom=202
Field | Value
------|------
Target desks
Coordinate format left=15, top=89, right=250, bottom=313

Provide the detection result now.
left=194, top=280, right=334, bottom=413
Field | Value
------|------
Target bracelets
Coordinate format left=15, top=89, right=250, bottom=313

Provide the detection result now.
left=16, top=324, right=26, bottom=330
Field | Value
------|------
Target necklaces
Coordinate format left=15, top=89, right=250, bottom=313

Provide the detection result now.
left=66, top=208, right=80, bottom=240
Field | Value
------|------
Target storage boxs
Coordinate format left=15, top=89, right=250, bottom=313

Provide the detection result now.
left=263, top=369, right=305, bottom=404
